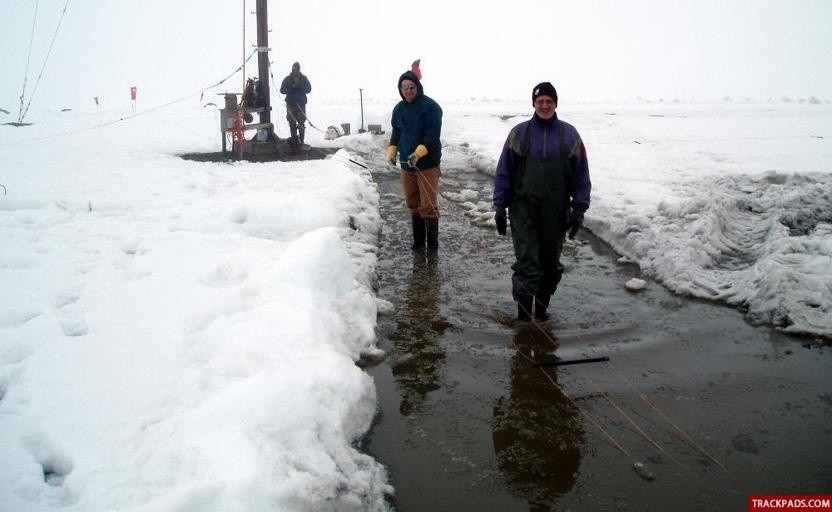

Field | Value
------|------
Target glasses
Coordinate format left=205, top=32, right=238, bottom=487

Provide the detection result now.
left=401, top=84, right=416, bottom=89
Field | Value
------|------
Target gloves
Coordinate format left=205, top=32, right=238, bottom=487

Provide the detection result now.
left=566, top=202, right=589, bottom=239
left=408, top=145, right=428, bottom=167
left=387, top=145, right=397, bottom=166
left=495, top=214, right=506, bottom=235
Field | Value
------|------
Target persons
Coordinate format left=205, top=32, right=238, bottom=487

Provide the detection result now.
left=280, top=62, right=311, bottom=148
left=493, top=82, right=591, bottom=322
left=411, top=58, right=422, bottom=80
left=387, top=71, right=442, bottom=249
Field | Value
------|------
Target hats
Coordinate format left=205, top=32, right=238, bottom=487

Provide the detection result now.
left=292, top=62, right=300, bottom=70
left=532, top=82, right=557, bottom=108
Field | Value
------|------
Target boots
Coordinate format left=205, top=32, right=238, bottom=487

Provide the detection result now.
left=291, top=128, right=304, bottom=145
left=535, top=294, right=550, bottom=318
left=411, top=213, right=426, bottom=249
left=518, top=296, right=532, bottom=322
left=425, top=217, right=438, bottom=243
